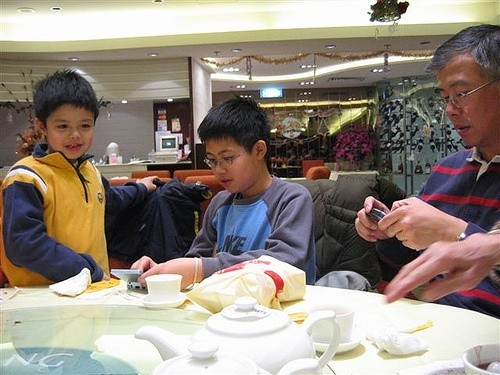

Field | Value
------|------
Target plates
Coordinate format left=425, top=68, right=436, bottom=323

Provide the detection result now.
left=139, top=292, right=186, bottom=309
left=309, top=338, right=364, bottom=354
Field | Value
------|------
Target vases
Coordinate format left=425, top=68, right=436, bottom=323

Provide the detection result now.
left=339, top=156, right=360, bottom=171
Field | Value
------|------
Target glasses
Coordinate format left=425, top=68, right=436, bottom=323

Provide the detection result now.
left=435, top=77, right=499, bottom=112
left=203, top=152, right=252, bottom=169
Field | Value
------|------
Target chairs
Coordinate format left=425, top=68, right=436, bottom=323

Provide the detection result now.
left=307, top=166, right=331, bottom=180
left=106, top=177, right=172, bottom=283
left=302, top=159, right=324, bottom=176
left=184, top=176, right=227, bottom=231
left=174, top=169, right=214, bottom=182
left=131, top=170, right=171, bottom=178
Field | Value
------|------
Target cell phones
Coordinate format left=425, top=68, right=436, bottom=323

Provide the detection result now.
left=111, top=268, right=144, bottom=282
left=366, top=208, right=386, bottom=224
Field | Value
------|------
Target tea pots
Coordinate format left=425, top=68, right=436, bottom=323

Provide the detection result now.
left=156, top=334, right=272, bottom=375
left=136, top=296, right=342, bottom=375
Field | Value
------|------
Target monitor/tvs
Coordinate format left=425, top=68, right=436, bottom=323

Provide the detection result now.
left=260, top=89, right=284, bottom=99
left=160, top=136, right=179, bottom=151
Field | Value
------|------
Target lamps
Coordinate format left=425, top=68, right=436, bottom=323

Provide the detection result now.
left=368, top=1, right=410, bottom=41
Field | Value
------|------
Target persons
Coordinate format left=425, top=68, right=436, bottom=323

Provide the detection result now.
left=385, top=221, right=500, bottom=305
left=0, top=70, right=162, bottom=287
left=131, top=94, right=316, bottom=287
left=356, top=24, right=500, bottom=320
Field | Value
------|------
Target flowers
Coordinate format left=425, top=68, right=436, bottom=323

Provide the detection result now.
left=15, top=124, right=45, bottom=157
left=334, top=127, right=375, bottom=162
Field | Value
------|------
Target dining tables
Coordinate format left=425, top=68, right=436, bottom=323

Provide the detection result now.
left=0, top=280, right=500, bottom=375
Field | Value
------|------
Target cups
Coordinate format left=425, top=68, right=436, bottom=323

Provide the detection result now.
left=464, top=343, right=499, bottom=375
left=145, top=274, right=183, bottom=302
left=308, top=304, right=354, bottom=345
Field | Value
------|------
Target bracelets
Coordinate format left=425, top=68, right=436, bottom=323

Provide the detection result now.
left=456, top=231, right=466, bottom=243
left=182, top=257, right=198, bottom=290
